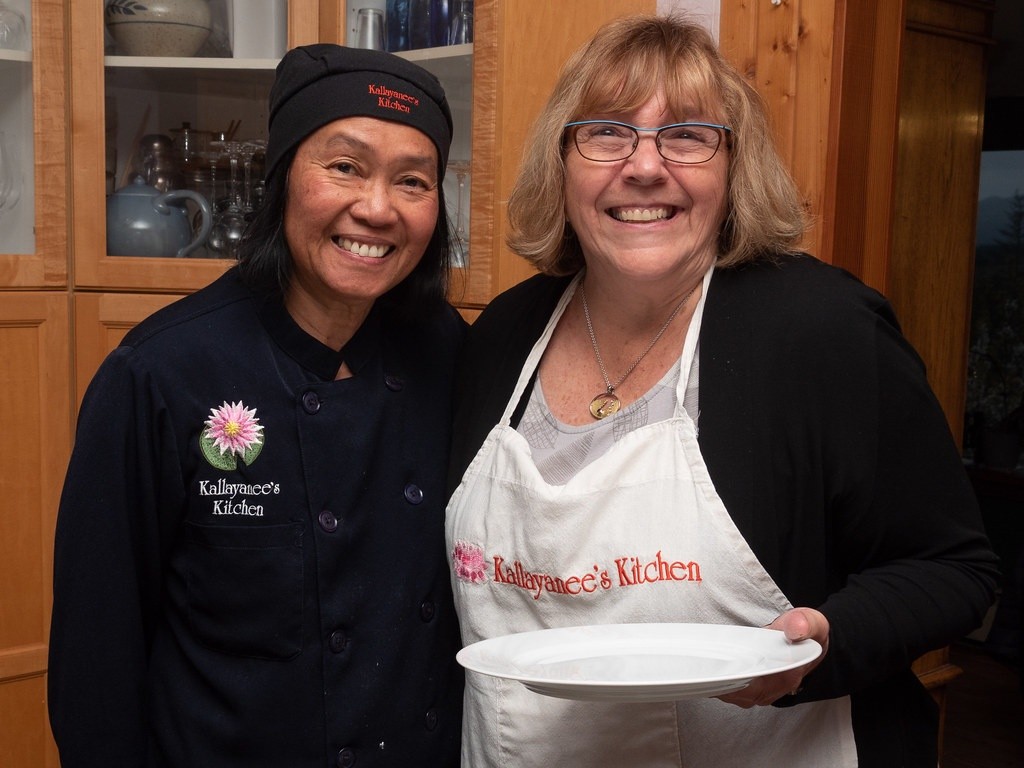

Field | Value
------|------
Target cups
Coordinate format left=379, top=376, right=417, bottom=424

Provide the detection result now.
left=353, top=8, right=386, bottom=54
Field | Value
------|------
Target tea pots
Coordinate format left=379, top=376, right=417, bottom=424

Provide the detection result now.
left=106, top=173, right=212, bottom=258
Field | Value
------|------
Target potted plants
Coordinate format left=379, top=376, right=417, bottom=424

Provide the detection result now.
left=968, top=253, right=1024, bottom=470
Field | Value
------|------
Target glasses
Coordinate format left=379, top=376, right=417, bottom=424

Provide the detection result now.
left=561, top=120, right=734, bottom=164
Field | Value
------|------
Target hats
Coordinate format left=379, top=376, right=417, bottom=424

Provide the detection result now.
left=265, top=44, right=452, bottom=190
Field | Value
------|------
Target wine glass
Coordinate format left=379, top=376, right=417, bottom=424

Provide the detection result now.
left=194, top=141, right=268, bottom=258
left=440, top=159, right=471, bottom=269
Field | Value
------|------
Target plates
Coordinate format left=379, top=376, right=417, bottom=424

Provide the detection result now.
left=456, top=623, right=822, bottom=702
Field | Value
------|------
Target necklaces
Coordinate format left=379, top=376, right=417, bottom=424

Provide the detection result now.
left=581, top=279, right=694, bottom=419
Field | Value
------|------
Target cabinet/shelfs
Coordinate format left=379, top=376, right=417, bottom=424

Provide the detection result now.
left=0, top=0, right=655, bottom=768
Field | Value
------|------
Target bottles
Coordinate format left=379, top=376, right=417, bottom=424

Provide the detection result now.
left=384, top=0, right=473, bottom=52
left=131, top=133, right=182, bottom=195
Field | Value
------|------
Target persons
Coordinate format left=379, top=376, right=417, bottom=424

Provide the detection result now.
left=46, top=43, right=472, bottom=768
left=444, top=16, right=1006, bottom=768
